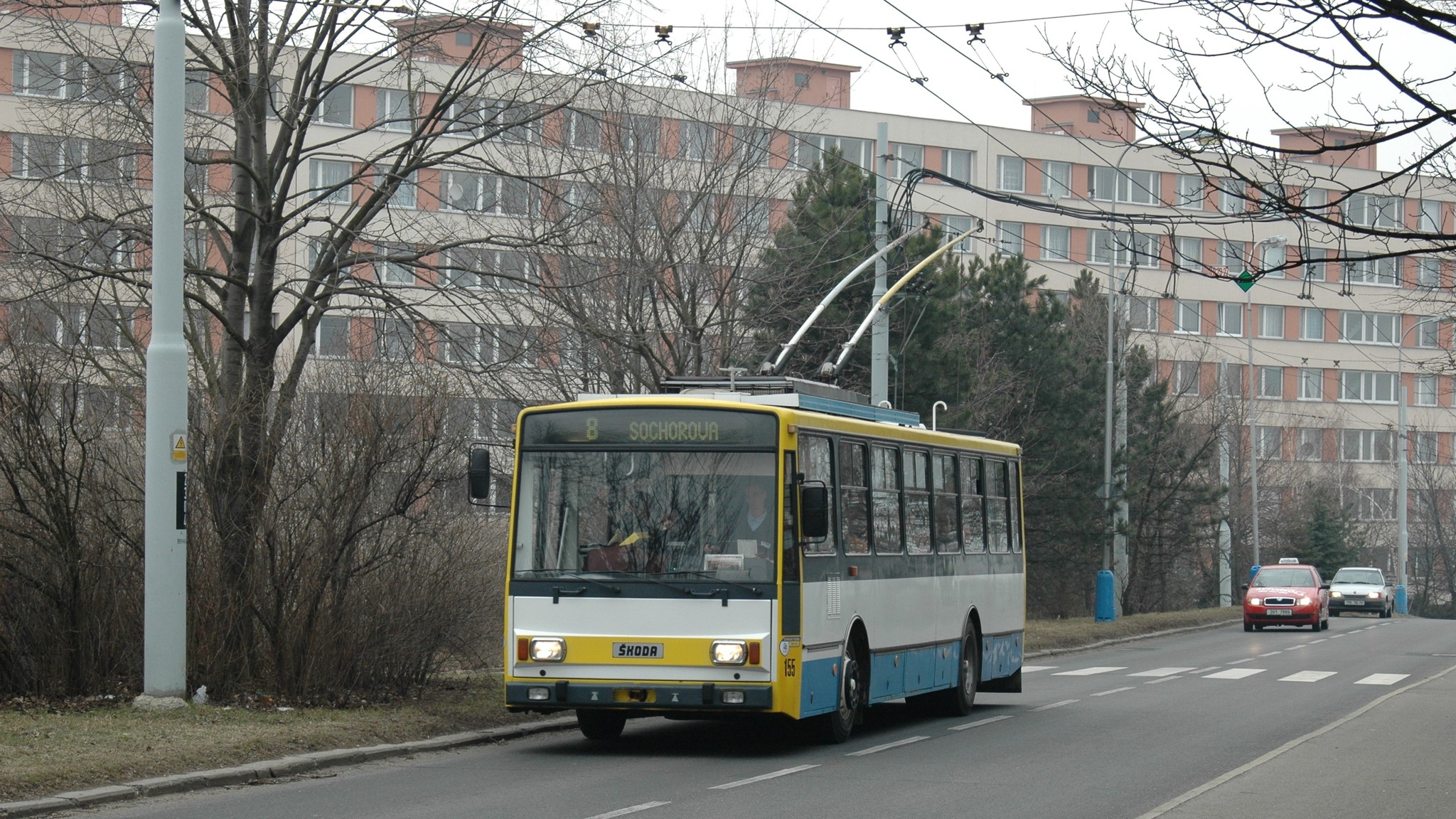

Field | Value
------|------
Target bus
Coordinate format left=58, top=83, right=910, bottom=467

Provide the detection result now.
left=467, top=218, right=1027, bottom=744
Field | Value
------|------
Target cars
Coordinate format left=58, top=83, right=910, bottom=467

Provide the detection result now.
left=1241, top=557, right=1330, bottom=632
left=1329, top=566, right=1394, bottom=618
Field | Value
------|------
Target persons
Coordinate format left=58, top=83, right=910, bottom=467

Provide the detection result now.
left=609, top=492, right=659, bottom=548
left=704, top=475, right=775, bottom=560
left=935, top=514, right=971, bottom=553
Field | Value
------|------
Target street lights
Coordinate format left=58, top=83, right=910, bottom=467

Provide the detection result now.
left=1397, top=312, right=1456, bottom=442
left=1248, top=237, right=1290, bottom=566
left=1102, top=131, right=1222, bottom=569
